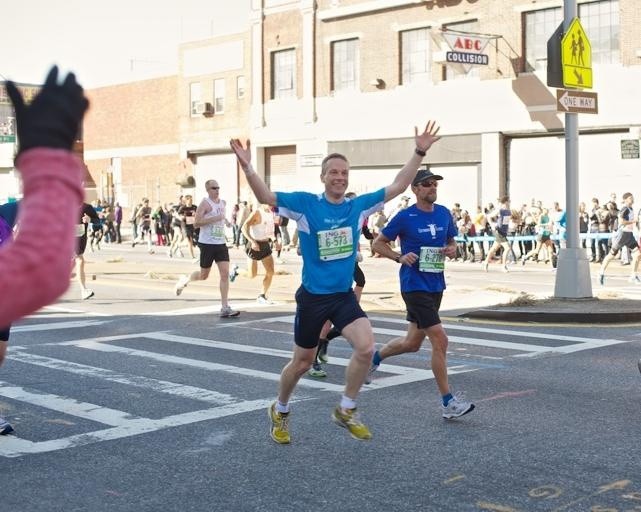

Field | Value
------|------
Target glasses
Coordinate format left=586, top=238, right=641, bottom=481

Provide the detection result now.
left=413, top=180, right=438, bottom=189
left=206, top=186, right=219, bottom=191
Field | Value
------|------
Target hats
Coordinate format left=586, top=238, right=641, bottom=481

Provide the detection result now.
left=411, top=168, right=444, bottom=188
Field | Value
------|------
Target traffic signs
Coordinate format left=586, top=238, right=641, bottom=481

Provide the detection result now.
left=553, top=88, right=599, bottom=116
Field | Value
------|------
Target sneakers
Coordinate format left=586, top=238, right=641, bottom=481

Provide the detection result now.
left=628, top=274, right=641, bottom=284
left=440, top=396, right=476, bottom=421
left=363, top=349, right=380, bottom=386
left=330, top=404, right=372, bottom=442
left=305, top=339, right=329, bottom=379
left=266, top=400, right=291, bottom=446
left=79, top=288, right=94, bottom=300
left=229, top=265, right=239, bottom=282
left=172, top=273, right=191, bottom=296
left=89, top=235, right=300, bottom=264
left=596, top=272, right=606, bottom=286
left=256, top=293, right=274, bottom=306
left=446, top=250, right=635, bottom=272
left=219, top=305, right=240, bottom=318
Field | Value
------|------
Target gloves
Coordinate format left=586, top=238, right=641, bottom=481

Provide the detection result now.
left=3, top=65, right=89, bottom=149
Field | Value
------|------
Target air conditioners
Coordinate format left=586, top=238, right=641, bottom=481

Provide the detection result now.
left=196, top=102, right=212, bottom=114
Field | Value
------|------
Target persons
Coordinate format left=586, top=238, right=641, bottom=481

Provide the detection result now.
left=0, top=201, right=19, bottom=435
left=231, top=121, right=442, bottom=446
left=230, top=121, right=475, bottom=447
left=74, top=179, right=474, bottom=319
left=0, top=65, right=89, bottom=330
left=469, top=196, right=566, bottom=273
left=580, top=192, right=641, bottom=286
left=365, top=171, right=475, bottom=418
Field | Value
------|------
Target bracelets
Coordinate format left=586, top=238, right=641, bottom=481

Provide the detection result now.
left=414, top=147, right=426, bottom=156
